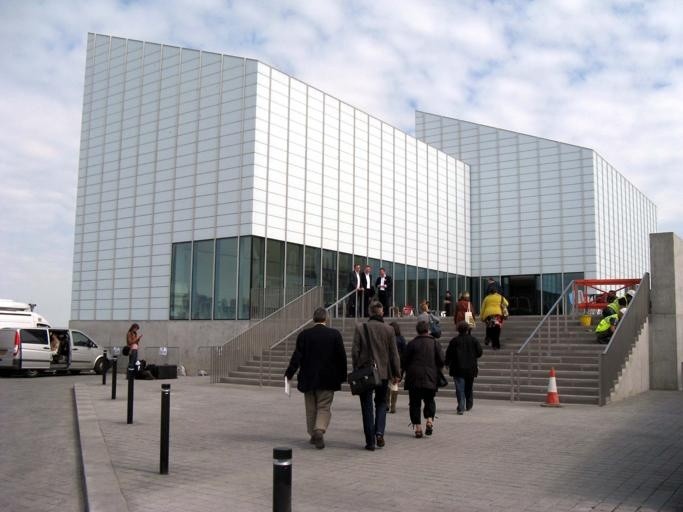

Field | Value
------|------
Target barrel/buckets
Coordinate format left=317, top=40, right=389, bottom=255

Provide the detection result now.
left=579, top=315, right=592, bottom=328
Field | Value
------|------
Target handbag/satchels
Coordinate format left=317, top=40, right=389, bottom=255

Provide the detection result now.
left=431, top=321, right=441, bottom=338
left=464, top=311, right=476, bottom=328
left=437, top=368, right=448, bottom=388
left=348, top=360, right=382, bottom=395
left=122, top=346, right=130, bottom=356
left=501, top=304, right=508, bottom=320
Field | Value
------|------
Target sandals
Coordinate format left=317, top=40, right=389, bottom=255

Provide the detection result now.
left=426, top=421, right=433, bottom=435
left=415, top=431, right=422, bottom=437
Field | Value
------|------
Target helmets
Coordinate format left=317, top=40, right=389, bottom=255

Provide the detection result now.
left=626, top=289, right=636, bottom=298
left=619, top=308, right=628, bottom=315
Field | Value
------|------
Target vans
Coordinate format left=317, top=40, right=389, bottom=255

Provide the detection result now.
left=0, top=327, right=114, bottom=377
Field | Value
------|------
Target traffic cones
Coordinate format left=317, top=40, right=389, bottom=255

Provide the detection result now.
left=541, top=366, right=563, bottom=407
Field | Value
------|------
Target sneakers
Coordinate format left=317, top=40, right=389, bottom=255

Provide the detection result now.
left=376, top=434, right=385, bottom=447
left=310, top=430, right=325, bottom=449
left=364, top=443, right=375, bottom=451
left=456, top=410, right=463, bottom=414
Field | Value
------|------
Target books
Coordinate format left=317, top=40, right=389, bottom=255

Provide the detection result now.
left=284, top=376, right=291, bottom=396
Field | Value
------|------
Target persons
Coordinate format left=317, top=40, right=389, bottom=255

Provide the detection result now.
left=348, top=264, right=393, bottom=318
left=595, top=290, right=634, bottom=344
left=50, top=334, right=60, bottom=357
left=444, top=321, right=482, bottom=414
left=352, top=301, right=403, bottom=450
left=485, top=278, right=497, bottom=296
left=126, top=324, right=144, bottom=378
left=387, top=300, right=447, bottom=439
left=479, top=287, right=510, bottom=349
left=444, top=290, right=453, bottom=318
left=455, top=292, right=476, bottom=335
left=282, top=307, right=348, bottom=448
